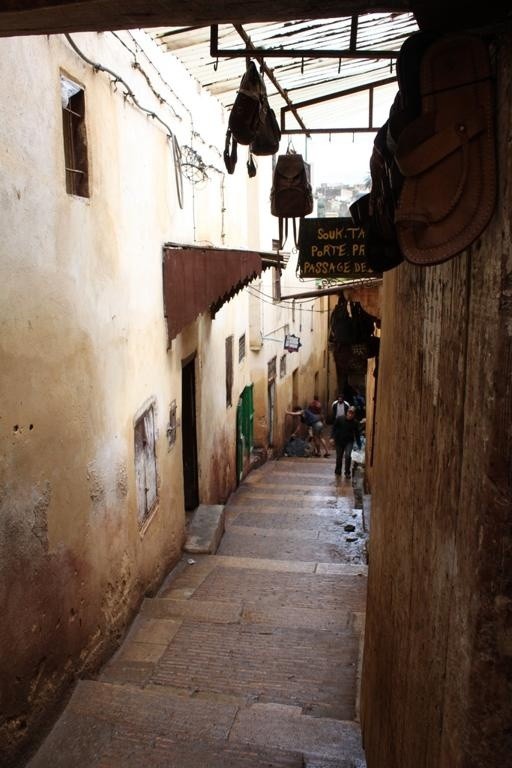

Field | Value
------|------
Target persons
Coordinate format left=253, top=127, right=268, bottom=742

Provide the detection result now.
left=308, top=395, right=328, bottom=429
left=285, top=406, right=332, bottom=457
left=329, top=395, right=350, bottom=445
left=330, top=405, right=361, bottom=479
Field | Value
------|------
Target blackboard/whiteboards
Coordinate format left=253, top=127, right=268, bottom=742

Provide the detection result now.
left=299, top=217, right=383, bottom=277
